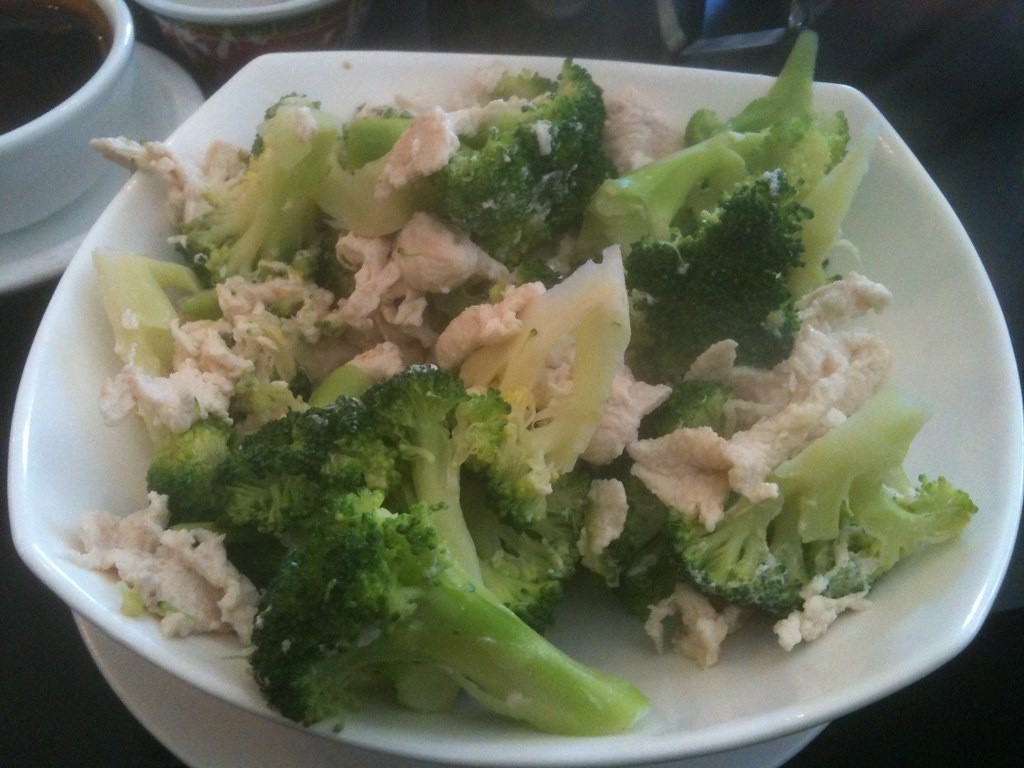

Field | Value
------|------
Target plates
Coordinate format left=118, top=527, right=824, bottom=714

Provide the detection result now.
left=0, top=40, right=212, bottom=292
left=74, top=609, right=836, bottom=768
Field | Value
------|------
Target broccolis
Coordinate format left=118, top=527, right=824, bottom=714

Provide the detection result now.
left=92, top=29, right=979, bottom=736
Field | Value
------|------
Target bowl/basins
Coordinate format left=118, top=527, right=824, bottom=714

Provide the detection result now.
left=8, top=52, right=1020, bottom=768
left=1, top=1, right=135, bottom=232
